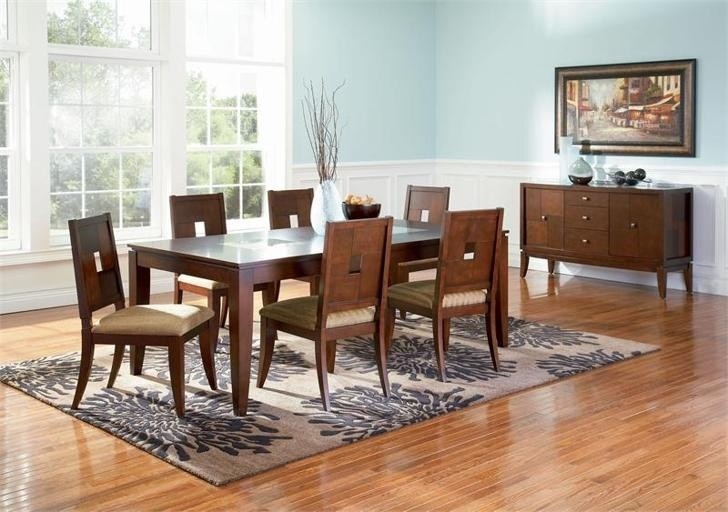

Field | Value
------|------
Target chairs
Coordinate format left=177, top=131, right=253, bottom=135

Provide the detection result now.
left=167, top=192, right=274, bottom=348
left=267, top=188, right=323, bottom=340
left=374, top=187, right=452, bottom=319
left=368, top=206, right=504, bottom=380
left=70, top=213, right=219, bottom=418
left=255, top=215, right=394, bottom=412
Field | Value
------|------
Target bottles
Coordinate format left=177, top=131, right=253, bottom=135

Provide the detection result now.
left=568, top=157, right=593, bottom=185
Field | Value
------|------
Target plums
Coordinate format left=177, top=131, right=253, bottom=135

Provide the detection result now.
left=615, top=169, right=646, bottom=185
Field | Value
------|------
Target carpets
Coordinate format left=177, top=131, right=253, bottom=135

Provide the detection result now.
left=1, top=306, right=662, bottom=486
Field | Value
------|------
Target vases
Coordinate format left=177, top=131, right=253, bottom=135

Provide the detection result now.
left=309, top=179, right=344, bottom=235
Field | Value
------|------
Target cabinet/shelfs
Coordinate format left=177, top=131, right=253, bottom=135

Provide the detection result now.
left=519, top=182, right=694, bottom=300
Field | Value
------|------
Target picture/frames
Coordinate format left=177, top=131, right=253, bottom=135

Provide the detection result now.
left=554, top=58, right=696, bottom=157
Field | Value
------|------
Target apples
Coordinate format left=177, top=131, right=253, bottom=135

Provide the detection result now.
left=346, top=193, right=373, bottom=205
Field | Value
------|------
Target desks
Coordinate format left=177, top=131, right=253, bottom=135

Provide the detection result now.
left=127, top=218, right=510, bottom=415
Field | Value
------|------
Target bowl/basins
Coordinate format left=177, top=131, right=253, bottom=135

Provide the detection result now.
left=341, top=202, right=381, bottom=222
